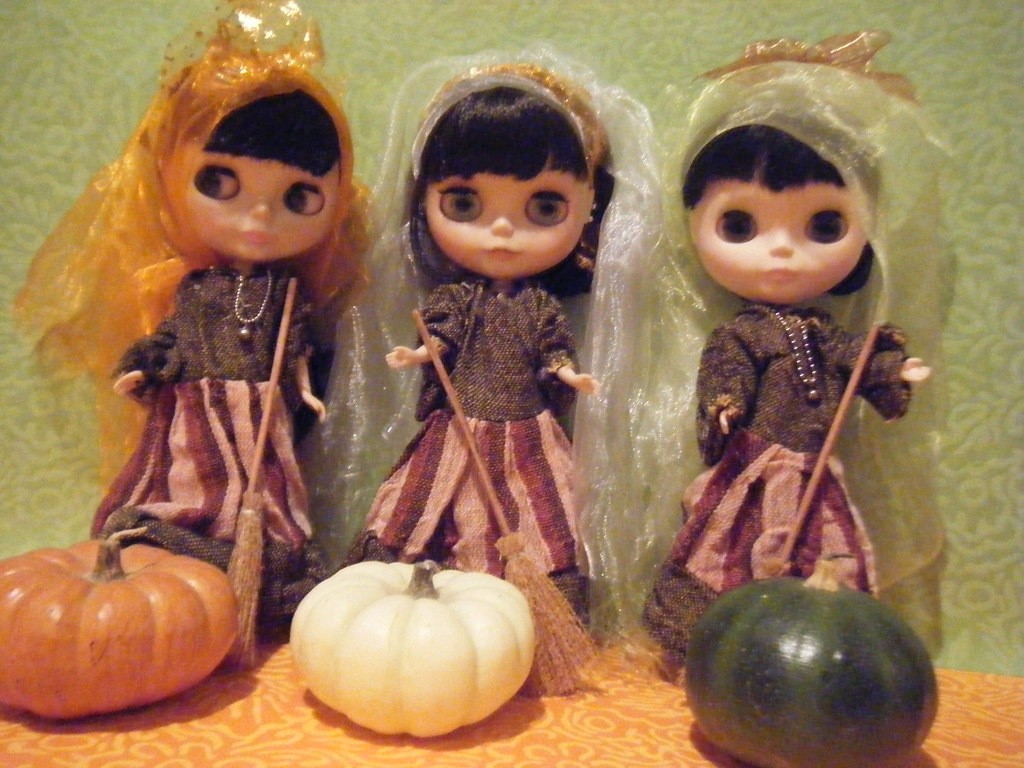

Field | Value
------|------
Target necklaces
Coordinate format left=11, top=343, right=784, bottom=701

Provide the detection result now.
left=776, top=311, right=820, bottom=404
left=235, top=267, right=272, bottom=339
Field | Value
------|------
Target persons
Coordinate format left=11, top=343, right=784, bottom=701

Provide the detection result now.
left=636, top=34, right=950, bottom=683
left=20, top=0, right=372, bottom=622
left=314, top=43, right=662, bottom=652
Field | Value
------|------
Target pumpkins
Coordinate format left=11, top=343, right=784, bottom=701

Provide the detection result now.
left=685, top=562, right=940, bottom=768
left=0, top=536, right=238, bottom=720
left=290, top=561, right=536, bottom=738
